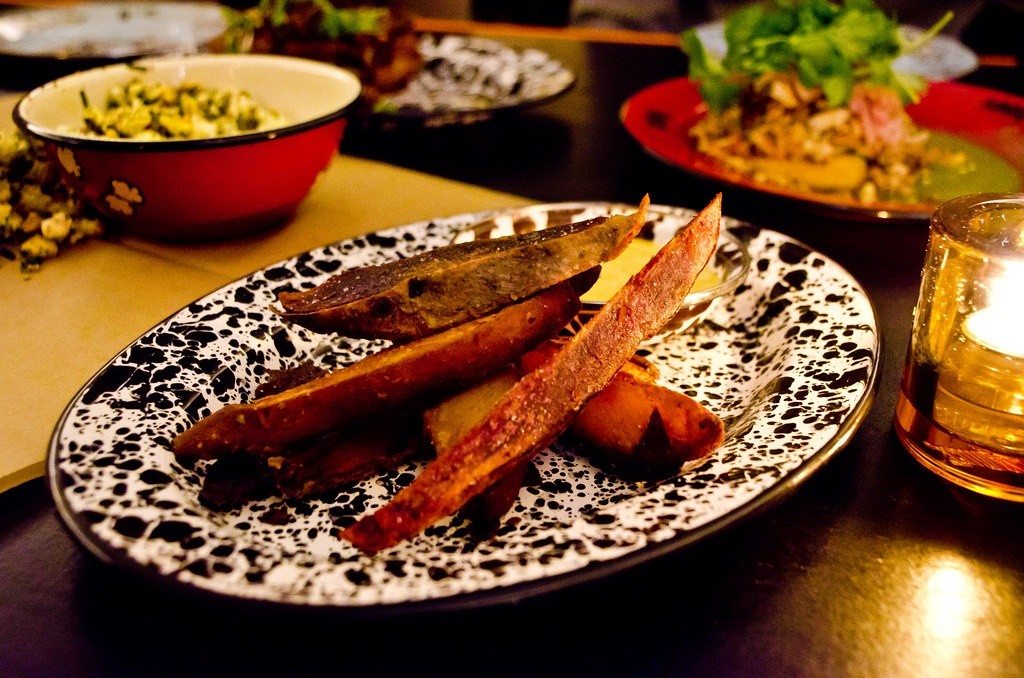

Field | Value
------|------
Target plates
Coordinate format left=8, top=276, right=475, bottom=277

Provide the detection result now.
left=693, top=19, right=980, bottom=83
left=0, top=1, right=244, bottom=86
left=619, top=75, right=1024, bottom=223
left=369, top=30, right=576, bottom=126
left=45, top=202, right=884, bottom=616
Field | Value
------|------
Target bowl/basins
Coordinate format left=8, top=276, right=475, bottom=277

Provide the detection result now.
left=12, top=53, right=363, bottom=242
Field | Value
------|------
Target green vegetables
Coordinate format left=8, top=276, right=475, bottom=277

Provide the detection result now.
left=679, top=0, right=956, bottom=116
left=221, top=0, right=386, bottom=56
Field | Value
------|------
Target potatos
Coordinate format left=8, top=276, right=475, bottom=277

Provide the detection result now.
left=171, top=190, right=733, bottom=552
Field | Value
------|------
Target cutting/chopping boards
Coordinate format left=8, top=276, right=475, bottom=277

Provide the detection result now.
left=0, top=90, right=541, bottom=500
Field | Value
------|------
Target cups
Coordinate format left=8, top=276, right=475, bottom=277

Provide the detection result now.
left=893, top=192, right=1024, bottom=503
